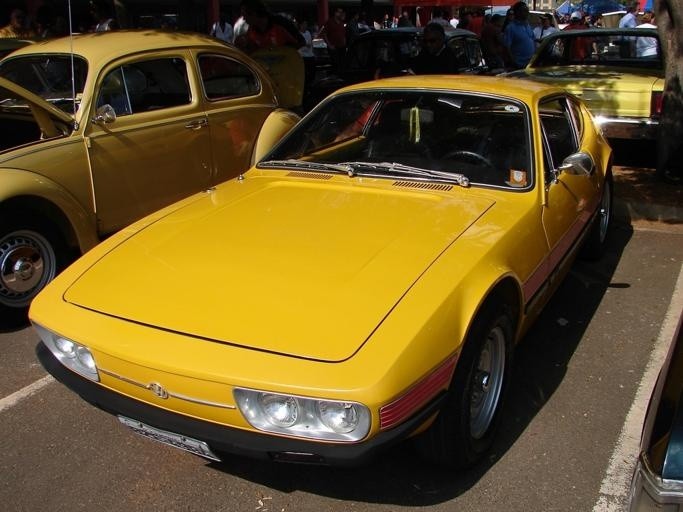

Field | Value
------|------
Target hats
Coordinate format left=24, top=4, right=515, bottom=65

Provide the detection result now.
left=538, top=14, right=552, bottom=19
left=571, top=11, right=582, bottom=20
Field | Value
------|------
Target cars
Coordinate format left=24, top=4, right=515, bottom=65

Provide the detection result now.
left=21, top=72, right=617, bottom=496
left=303, top=25, right=491, bottom=123
left=0, top=23, right=319, bottom=321
left=507, top=28, right=675, bottom=147
left=635, top=305, right=680, bottom=509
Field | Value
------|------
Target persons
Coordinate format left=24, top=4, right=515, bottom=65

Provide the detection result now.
left=456, top=9, right=515, bottom=73
left=427, top=11, right=451, bottom=27
left=551, top=9, right=603, bottom=28
left=345, top=11, right=361, bottom=50
left=618, top=2, right=640, bottom=59
left=628, top=11, right=658, bottom=60
left=234, top=3, right=306, bottom=54
left=560, top=11, right=597, bottom=64
left=415, top=22, right=459, bottom=74
left=323, top=8, right=348, bottom=68
left=297, top=20, right=316, bottom=83
left=532, top=13, right=559, bottom=55
left=209, top=14, right=234, bottom=43
left=497, top=2, right=537, bottom=71
left=2, top=7, right=40, bottom=39
